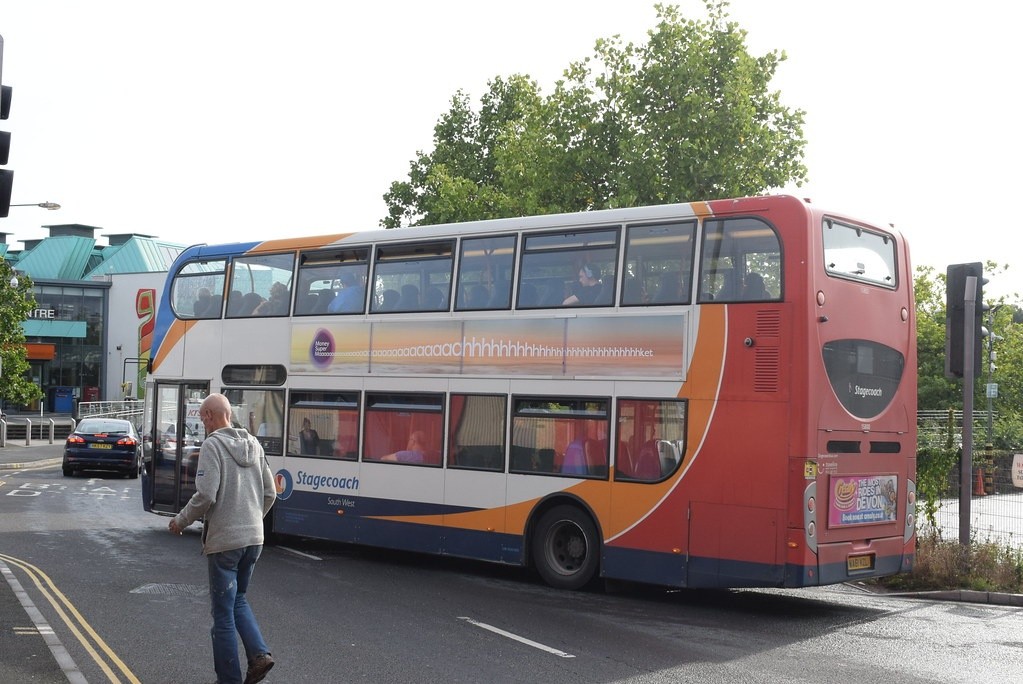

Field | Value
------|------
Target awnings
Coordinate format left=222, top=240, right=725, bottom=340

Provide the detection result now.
left=20, top=319, right=87, bottom=338
left=3, top=343, right=56, bottom=360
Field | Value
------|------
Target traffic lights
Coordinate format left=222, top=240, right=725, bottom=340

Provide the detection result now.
left=945, top=261, right=988, bottom=379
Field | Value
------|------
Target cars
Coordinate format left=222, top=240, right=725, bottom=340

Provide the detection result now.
left=61, top=418, right=143, bottom=480
left=136, top=419, right=200, bottom=463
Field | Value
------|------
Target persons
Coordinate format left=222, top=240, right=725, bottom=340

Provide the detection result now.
left=299, top=418, right=321, bottom=456
left=328, top=273, right=364, bottom=313
left=193, top=287, right=213, bottom=318
left=380, top=430, right=426, bottom=466
left=169, top=394, right=277, bottom=684
left=562, top=263, right=602, bottom=306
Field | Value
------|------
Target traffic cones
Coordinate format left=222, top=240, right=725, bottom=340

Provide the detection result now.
left=973, top=468, right=988, bottom=496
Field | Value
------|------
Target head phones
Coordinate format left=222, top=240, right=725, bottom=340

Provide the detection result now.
left=583, top=264, right=593, bottom=277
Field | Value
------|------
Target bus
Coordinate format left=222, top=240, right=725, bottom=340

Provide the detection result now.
left=140, top=195, right=919, bottom=592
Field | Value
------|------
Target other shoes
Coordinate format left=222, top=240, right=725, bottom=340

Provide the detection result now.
left=243, top=654, right=275, bottom=684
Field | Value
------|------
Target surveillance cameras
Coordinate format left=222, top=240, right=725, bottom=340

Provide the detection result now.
left=10, top=277, right=18, bottom=289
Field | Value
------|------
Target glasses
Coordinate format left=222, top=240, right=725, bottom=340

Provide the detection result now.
left=407, top=438, right=416, bottom=442
left=201, top=413, right=214, bottom=427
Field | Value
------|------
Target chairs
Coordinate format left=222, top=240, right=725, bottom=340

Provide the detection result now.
left=560, top=437, right=683, bottom=481
left=198, top=271, right=764, bottom=318
